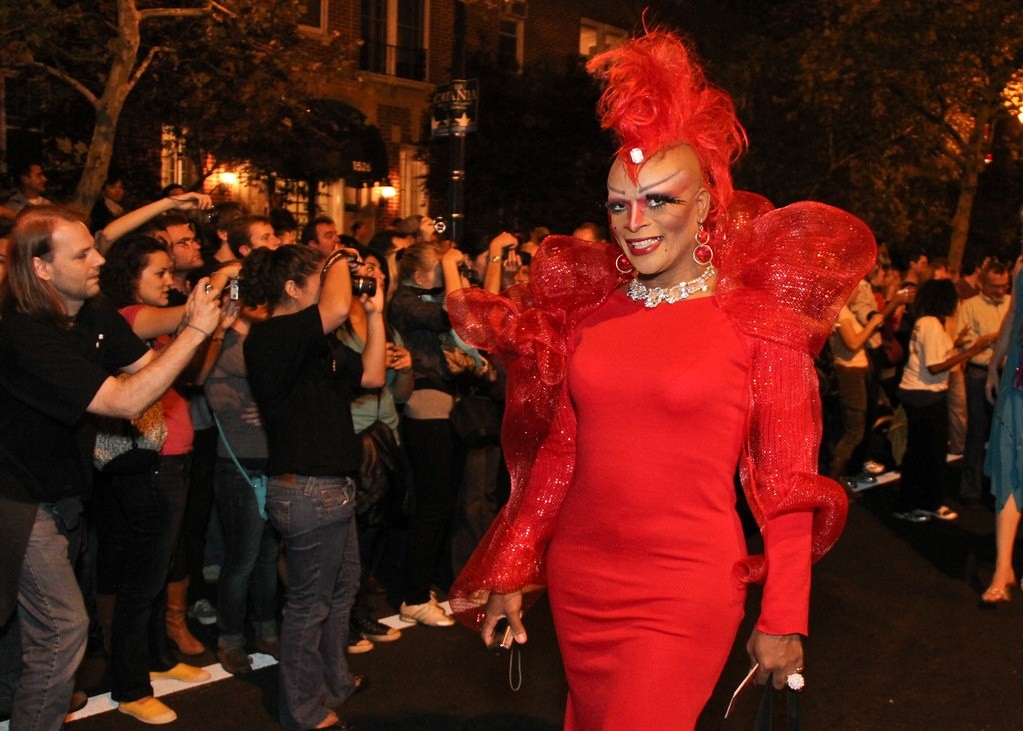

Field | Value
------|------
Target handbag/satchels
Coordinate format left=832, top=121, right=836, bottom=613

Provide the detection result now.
left=251, top=475, right=269, bottom=518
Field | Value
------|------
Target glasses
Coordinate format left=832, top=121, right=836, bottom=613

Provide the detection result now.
left=171, top=235, right=203, bottom=250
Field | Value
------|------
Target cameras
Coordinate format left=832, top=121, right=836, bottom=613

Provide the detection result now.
left=206, top=284, right=212, bottom=294
left=456, top=262, right=479, bottom=285
left=348, top=265, right=377, bottom=297
left=490, top=610, right=524, bottom=650
left=502, top=245, right=532, bottom=267
left=203, top=205, right=220, bottom=224
left=223, top=277, right=238, bottom=301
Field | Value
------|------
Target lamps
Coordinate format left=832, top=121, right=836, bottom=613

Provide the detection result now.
left=222, top=167, right=236, bottom=197
left=379, top=178, right=395, bottom=206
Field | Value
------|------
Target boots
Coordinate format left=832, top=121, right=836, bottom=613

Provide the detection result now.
left=94, top=592, right=116, bottom=656
left=165, top=580, right=205, bottom=656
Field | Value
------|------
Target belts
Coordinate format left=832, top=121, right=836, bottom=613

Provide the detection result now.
left=967, top=362, right=1004, bottom=375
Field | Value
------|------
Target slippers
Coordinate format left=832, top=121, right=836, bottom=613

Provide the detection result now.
left=982, top=577, right=1017, bottom=604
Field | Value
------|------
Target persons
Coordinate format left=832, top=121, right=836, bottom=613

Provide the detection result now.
left=448, top=5, right=878, bottom=731
left=0, top=157, right=1023, bottom=731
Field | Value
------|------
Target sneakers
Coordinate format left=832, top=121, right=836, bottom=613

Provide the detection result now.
left=187, top=597, right=217, bottom=625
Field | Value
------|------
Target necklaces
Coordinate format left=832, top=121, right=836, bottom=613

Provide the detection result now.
left=626, top=264, right=716, bottom=308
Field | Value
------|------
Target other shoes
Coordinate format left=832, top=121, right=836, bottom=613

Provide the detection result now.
left=217, top=634, right=252, bottom=675
left=307, top=710, right=348, bottom=731
left=945, top=453, right=964, bottom=464
left=346, top=628, right=375, bottom=654
left=864, top=460, right=885, bottom=474
left=119, top=698, right=177, bottom=724
left=892, top=509, right=930, bottom=522
left=823, top=466, right=857, bottom=489
left=66, top=691, right=87, bottom=714
left=400, top=590, right=456, bottom=627
left=847, top=466, right=877, bottom=484
left=353, top=673, right=368, bottom=694
left=916, top=505, right=958, bottom=520
left=350, top=605, right=401, bottom=642
left=254, top=619, right=282, bottom=660
left=148, top=662, right=211, bottom=682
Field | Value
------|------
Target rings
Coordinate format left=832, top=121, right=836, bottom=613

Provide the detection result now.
left=794, top=668, right=804, bottom=670
left=785, top=673, right=806, bottom=690
left=475, top=610, right=486, bottom=622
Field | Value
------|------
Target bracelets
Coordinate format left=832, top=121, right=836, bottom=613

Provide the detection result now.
left=188, top=324, right=209, bottom=337
left=489, top=257, right=501, bottom=263
